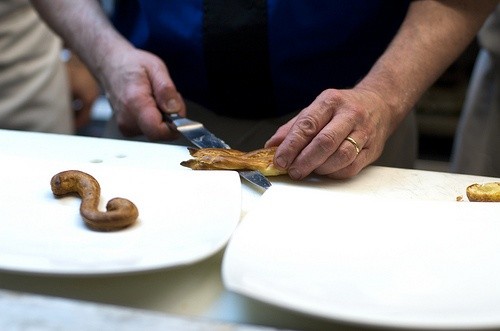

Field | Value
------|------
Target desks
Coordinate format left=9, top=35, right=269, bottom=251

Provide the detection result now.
left=1, top=133, right=499, bottom=331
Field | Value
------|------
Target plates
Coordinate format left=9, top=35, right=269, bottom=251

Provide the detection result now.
left=0, top=127, right=242, bottom=277
left=219, top=164, right=500, bottom=329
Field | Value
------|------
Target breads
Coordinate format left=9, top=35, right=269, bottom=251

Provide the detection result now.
left=50, top=171, right=139, bottom=229
left=178, top=145, right=296, bottom=178
left=465, top=181, right=500, bottom=202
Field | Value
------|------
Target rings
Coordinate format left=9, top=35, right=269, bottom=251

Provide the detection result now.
left=345, top=136, right=360, bottom=156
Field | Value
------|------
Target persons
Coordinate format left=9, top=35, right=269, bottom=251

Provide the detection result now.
left=27, top=0, right=500, bottom=180
left=0, top=0, right=101, bottom=135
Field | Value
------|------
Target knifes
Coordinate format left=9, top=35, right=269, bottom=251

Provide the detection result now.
left=167, top=111, right=272, bottom=189
